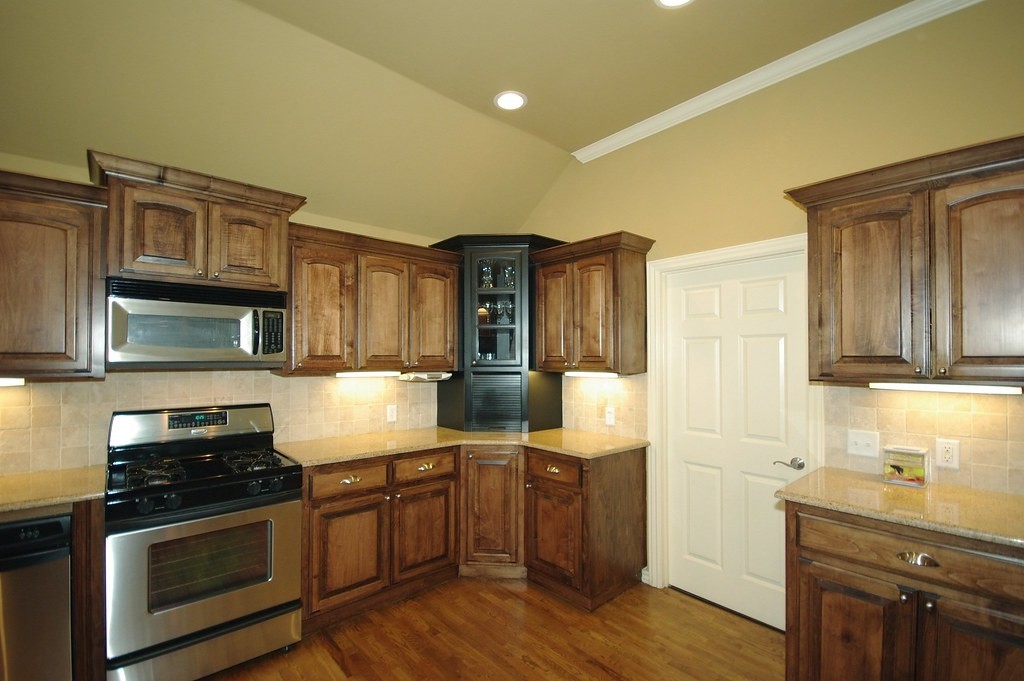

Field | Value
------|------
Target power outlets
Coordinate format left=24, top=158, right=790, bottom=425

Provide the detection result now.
left=935, top=439, right=960, bottom=470
left=605, top=407, right=615, bottom=426
left=387, top=405, right=397, bottom=422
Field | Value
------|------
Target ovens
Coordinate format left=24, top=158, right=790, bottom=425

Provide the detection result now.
left=107, top=497, right=302, bottom=681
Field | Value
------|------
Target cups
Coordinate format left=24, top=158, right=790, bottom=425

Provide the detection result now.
left=480, top=353, right=494, bottom=360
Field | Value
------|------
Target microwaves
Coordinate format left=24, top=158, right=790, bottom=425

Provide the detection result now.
left=102, top=276, right=287, bottom=378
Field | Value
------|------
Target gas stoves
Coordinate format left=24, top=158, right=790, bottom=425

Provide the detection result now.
left=102, top=402, right=300, bottom=492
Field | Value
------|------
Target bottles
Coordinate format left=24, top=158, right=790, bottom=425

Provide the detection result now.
left=504, top=265, right=515, bottom=288
left=481, top=267, right=493, bottom=288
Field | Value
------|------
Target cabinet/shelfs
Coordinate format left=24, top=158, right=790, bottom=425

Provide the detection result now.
left=524, top=445, right=647, bottom=610
left=527, top=230, right=657, bottom=375
left=0, top=169, right=106, bottom=380
left=785, top=499, right=1024, bottom=681
left=459, top=445, right=528, bottom=581
left=357, top=234, right=463, bottom=374
left=300, top=444, right=459, bottom=636
left=269, top=221, right=357, bottom=377
left=86, top=149, right=307, bottom=295
left=784, top=131, right=1024, bottom=388
left=427, top=234, right=573, bottom=432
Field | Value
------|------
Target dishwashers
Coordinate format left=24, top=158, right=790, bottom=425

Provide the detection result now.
left=0, top=512, right=75, bottom=681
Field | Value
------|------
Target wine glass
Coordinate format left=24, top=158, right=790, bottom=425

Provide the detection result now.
left=505, top=301, right=515, bottom=324
left=493, top=301, right=504, bottom=325
left=481, top=302, right=493, bottom=325
